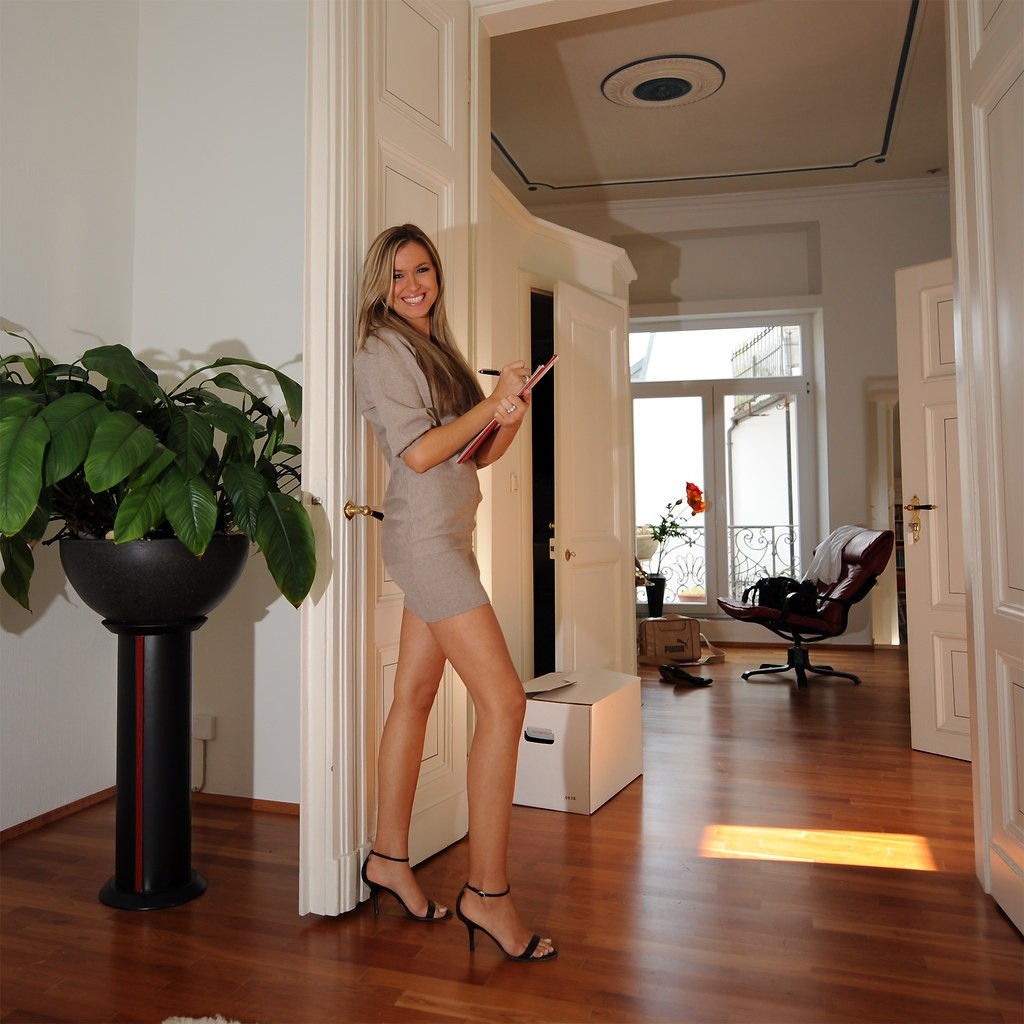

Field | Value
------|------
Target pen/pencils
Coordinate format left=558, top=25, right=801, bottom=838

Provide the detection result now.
left=478, top=368, right=501, bottom=377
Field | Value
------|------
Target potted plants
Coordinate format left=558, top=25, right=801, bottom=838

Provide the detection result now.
left=0, top=325, right=318, bottom=625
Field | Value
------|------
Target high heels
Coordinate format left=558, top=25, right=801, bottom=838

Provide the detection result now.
left=456, top=882, right=557, bottom=961
left=362, top=849, right=452, bottom=921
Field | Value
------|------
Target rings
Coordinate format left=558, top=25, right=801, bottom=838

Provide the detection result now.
left=506, top=405, right=517, bottom=414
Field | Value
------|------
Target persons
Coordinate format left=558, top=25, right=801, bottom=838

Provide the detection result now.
left=344, top=223, right=560, bottom=965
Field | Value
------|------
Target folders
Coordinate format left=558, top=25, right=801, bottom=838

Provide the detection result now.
left=456, top=354, right=559, bottom=465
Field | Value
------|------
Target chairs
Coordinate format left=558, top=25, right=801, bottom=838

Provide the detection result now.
left=717, top=524, right=895, bottom=690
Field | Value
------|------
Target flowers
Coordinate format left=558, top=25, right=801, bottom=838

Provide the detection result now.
left=636, top=524, right=652, bottom=535
left=650, top=480, right=710, bottom=574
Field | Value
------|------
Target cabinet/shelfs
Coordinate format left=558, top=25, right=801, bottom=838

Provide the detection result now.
left=862, top=375, right=908, bottom=649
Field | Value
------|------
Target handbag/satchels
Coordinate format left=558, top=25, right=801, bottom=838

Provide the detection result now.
left=741, top=577, right=818, bottom=623
left=638, top=614, right=727, bottom=667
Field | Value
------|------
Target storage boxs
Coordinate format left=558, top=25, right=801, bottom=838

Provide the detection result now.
left=512, top=666, right=645, bottom=818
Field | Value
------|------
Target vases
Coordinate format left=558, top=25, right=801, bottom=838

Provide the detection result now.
left=636, top=535, right=659, bottom=559
left=645, top=573, right=667, bottom=617
